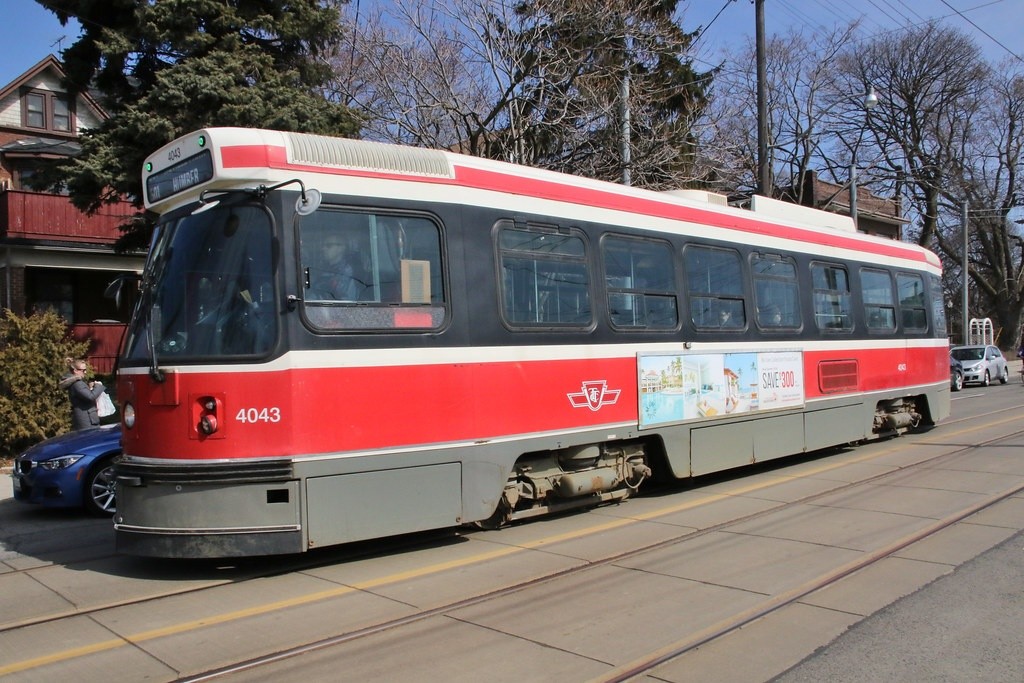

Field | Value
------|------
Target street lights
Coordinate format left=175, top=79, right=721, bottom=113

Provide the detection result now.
left=757, top=87, right=878, bottom=196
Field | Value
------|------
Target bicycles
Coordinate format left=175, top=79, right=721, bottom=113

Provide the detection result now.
left=1018, top=355, right=1024, bottom=384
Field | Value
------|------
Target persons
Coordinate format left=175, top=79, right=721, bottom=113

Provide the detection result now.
left=759, top=303, right=786, bottom=326
left=59, top=359, right=107, bottom=432
left=307, top=234, right=359, bottom=300
left=703, top=300, right=737, bottom=326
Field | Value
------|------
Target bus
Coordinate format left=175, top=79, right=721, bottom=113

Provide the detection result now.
left=109, top=124, right=952, bottom=557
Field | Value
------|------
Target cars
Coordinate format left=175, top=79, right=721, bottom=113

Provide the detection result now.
left=949, top=355, right=965, bottom=391
left=948, top=345, right=1009, bottom=387
left=9, top=421, right=124, bottom=518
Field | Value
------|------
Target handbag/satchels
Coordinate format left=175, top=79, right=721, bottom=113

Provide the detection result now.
left=95, top=391, right=116, bottom=418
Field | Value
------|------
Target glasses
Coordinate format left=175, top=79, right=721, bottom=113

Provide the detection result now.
left=76, top=369, right=86, bottom=373
left=322, top=243, right=345, bottom=249
